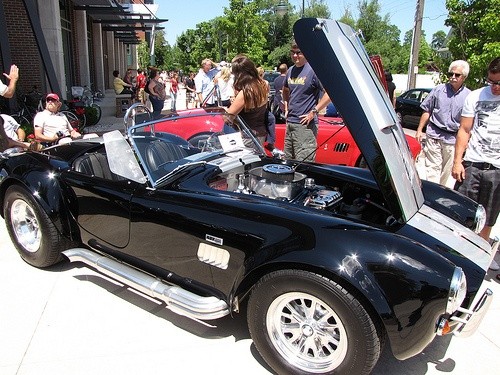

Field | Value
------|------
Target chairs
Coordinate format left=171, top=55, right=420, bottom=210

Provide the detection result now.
left=145, top=139, right=188, bottom=180
left=71, top=152, right=128, bottom=183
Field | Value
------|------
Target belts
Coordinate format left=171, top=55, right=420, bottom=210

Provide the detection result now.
left=472, top=163, right=499, bottom=170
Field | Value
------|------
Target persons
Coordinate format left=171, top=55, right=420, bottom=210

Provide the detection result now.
left=194, top=59, right=234, bottom=110
left=256, top=64, right=290, bottom=155
left=147, top=69, right=166, bottom=115
left=281, top=41, right=330, bottom=161
left=228, top=56, right=270, bottom=148
left=450, top=56, right=499, bottom=270
left=0, top=64, right=20, bottom=99
left=385, top=70, right=396, bottom=110
left=113, top=68, right=146, bottom=112
left=185, top=72, right=197, bottom=110
left=34, top=94, right=82, bottom=149
left=0, top=113, right=26, bottom=178
left=416, top=60, right=475, bottom=187
left=169, top=73, right=180, bottom=111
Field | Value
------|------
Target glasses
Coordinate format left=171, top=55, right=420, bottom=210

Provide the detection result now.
left=486, top=78, right=500, bottom=85
left=290, top=50, right=303, bottom=54
left=47, top=98, right=57, bottom=102
left=447, top=72, right=463, bottom=77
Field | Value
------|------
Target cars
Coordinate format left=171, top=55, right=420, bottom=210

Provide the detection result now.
left=261, top=70, right=281, bottom=100
left=0, top=18, right=500, bottom=375
left=394, top=88, right=434, bottom=133
left=146, top=55, right=422, bottom=173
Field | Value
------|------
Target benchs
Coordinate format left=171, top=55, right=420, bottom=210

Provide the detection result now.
left=116, top=93, right=133, bottom=118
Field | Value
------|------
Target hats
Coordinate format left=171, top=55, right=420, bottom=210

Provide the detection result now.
left=220, top=61, right=227, bottom=66
left=45, top=93, right=59, bottom=100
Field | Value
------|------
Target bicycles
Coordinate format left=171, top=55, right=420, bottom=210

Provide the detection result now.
left=7, top=85, right=102, bottom=134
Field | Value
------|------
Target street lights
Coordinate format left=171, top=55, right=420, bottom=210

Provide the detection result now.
left=278, top=0, right=305, bottom=19
left=206, top=30, right=222, bottom=62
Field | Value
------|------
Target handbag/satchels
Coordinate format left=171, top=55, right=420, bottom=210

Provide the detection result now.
left=144, top=81, right=155, bottom=94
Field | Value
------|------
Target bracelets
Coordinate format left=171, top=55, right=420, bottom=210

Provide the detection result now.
left=312, top=107, right=319, bottom=114
